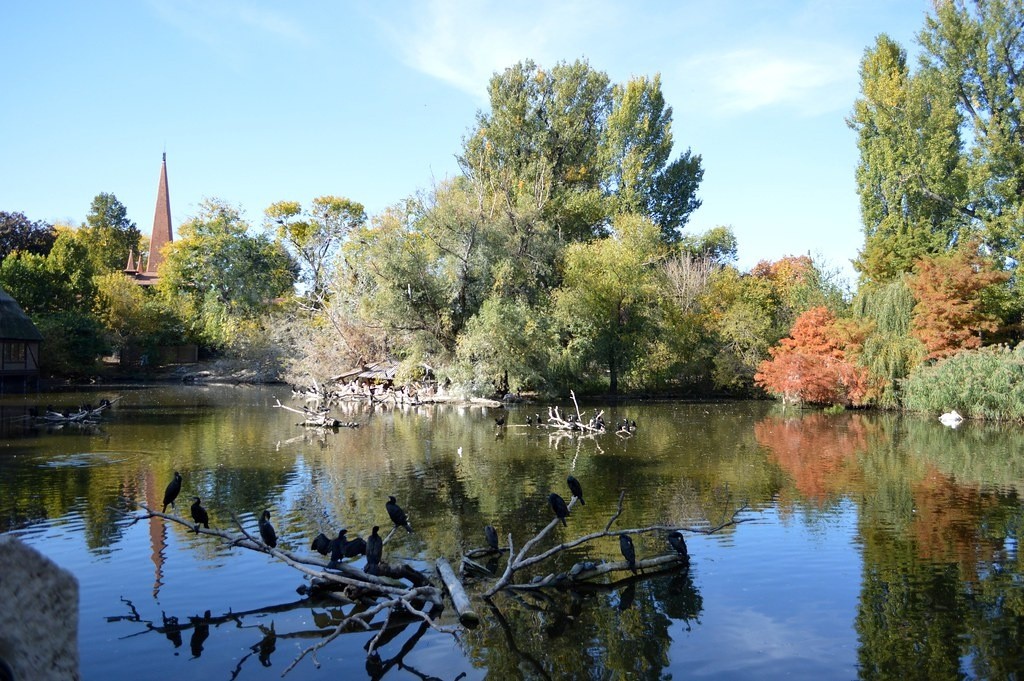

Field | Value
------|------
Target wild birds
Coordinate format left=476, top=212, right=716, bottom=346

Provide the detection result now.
left=668, top=531, right=688, bottom=559
left=385, top=495, right=414, bottom=533
left=551, top=408, right=605, bottom=431
left=618, top=534, right=638, bottom=575
left=550, top=492, right=571, bottom=528
left=258, top=509, right=278, bottom=548
left=615, top=418, right=637, bottom=432
left=28, top=398, right=112, bottom=420
left=190, top=496, right=210, bottom=529
left=311, top=525, right=385, bottom=573
left=525, top=412, right=543, bottom=425
left=494, top=414, right=505, bottom=426
left=162, top=471, right=183, bottom=514
left=485, top=524, right=505, bottom=556
left=567, top=475, right=586, bottom=506
